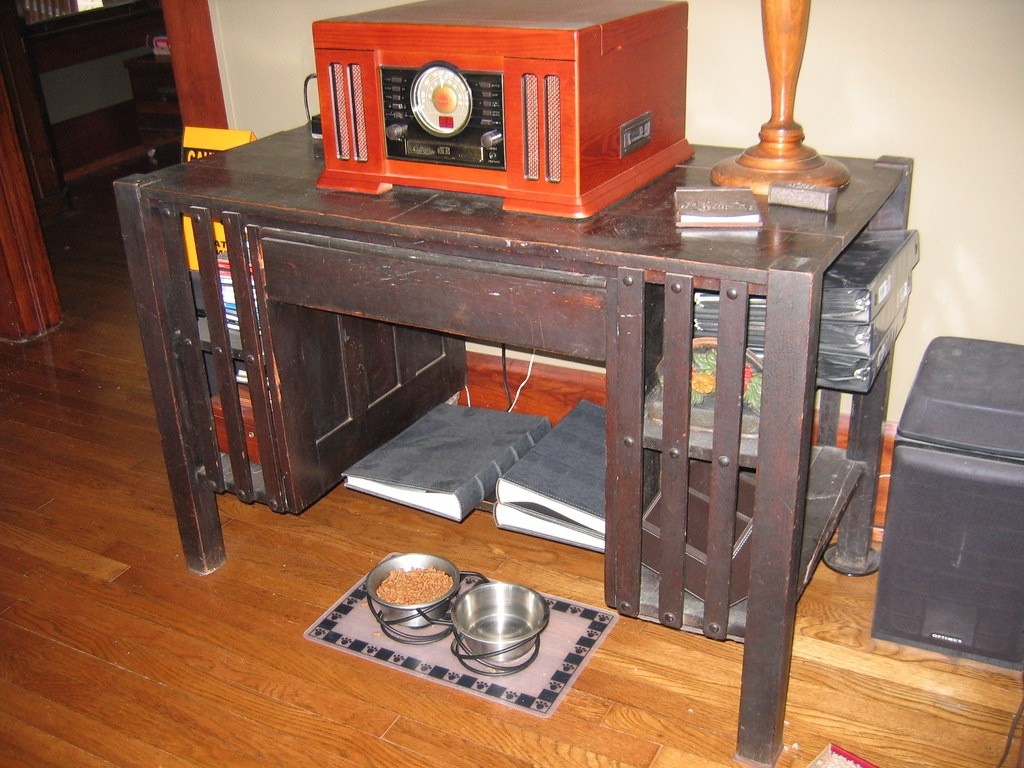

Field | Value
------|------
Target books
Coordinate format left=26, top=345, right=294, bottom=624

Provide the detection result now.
left=674, top=185, right=763, bottom=228
left=339, top=398, right=660, bottom=554
left=217, top=232, right=264, bottom=336
left=676, top=226, right=762, bottom=245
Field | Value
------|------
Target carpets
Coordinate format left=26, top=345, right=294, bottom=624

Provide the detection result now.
left=302, top=551, right=620, bottom=719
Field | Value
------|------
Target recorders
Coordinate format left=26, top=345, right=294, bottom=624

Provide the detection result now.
left=316, top=0, right=694, bottom=219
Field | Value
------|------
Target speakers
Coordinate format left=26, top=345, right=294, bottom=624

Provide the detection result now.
left=869, top=335, right=1023, bottom=674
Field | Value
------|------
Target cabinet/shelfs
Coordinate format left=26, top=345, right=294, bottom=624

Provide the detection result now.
left=122, top=52, right=184, bottom=151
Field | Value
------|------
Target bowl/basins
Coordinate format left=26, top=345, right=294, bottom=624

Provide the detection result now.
left=366, top=553, right=460, bottom=628
left=451, top=581, right=550, bottom=662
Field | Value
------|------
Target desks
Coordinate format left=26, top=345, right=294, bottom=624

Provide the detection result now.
left=110, top=122, right=916, bottom=768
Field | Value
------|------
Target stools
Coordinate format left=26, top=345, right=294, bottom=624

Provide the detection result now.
left=870, top=335, right=1024, bottom=672
